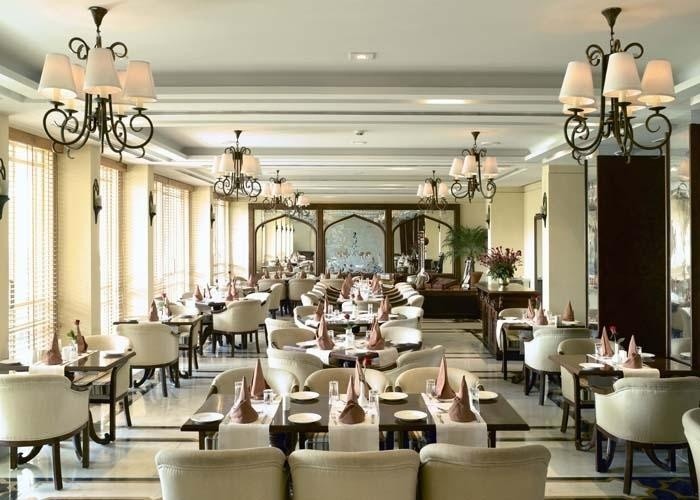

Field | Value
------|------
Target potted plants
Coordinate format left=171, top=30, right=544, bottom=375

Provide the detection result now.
left=439, top=220, right=490, bottom=288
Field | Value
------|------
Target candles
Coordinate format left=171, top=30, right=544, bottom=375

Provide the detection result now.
left=0, top=178, right=11, bottom=196
left=151, top=205, right=157, bottom=213
left=95, top=196, right=102, bottom=206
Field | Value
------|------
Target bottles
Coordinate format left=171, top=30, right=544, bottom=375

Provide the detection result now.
left=156, top=304, right=170, bottom=323
left=63, top=345, right=77, bottom=361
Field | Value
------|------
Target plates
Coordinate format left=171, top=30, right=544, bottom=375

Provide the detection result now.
left=190, top=412, right=224, bottom=423
left=380, top=399, right=408, bottom=406
left=480, top=399, right=498, bottom=405
left=394, top=418, right=426, bottom=426
left=288, top=412, right=322, bottom=424
left=380, top=391, right=409, bottom=402
left=642, top=352, right=655, bottom=358
left=289, top=391, right=320, bottom=400
left=642, top=358, right=655, bottom=362
left=472, top=390, right=499, bottom=400
left=579, top=362, right=604, bottom=369
left=394, top=410, right=427, bottom=422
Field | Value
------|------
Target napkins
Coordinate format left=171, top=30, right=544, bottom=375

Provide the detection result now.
left=448, top=375, right=479, bottom=425
left=622, top=333, right=645, bottom=371
left=430, top=356, right=458, bottom=402
left=39, top=331, right=63, bottom=369
left=226, top=375, right=262, bottom=427
left=351, top=353, right=375, bottom=402
left=336, top=376, right=369, bottom=428
left=597, top=325, right=616, bottom=359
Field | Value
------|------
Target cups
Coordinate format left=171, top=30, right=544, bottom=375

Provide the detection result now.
left=635, top=346, right=642, bottom=356
left=521, top=307, right=553, bottom=321
left=368, top=390, right=379, bottom=410
left=328, top=328, right=371, bottom=348
left=233, top=381, right=243, bottom=400
left=329, top=381, right=339, bottom=404
left=327, top=303, right=374, bottom=319
left=469, top=387, right=480, bottom=415
left=425, top=379, right=436, bottom=394
left=263, top=390, right=274, bottom=404
left=594, top=342, right=602, bottom=356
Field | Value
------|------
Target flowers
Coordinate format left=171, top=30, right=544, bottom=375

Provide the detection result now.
left=478, top=244, right=524, bottom=282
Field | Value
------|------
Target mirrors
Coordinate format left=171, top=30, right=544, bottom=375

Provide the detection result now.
left=395, top=216, right=456, bottom=280
left=256, top=214, right=318, bottom=278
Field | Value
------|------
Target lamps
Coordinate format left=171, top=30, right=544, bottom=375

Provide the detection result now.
left=289, top=190, right=312, bottom=219
left=259, top=169, right=296, bottom=213
left=210, top=131, right=264, bottom=204
left=36, top=6, right=158, bottom=164
left=416, top=168, right=452, bottom=214
left=447, top=131, right=498, bottom=206
left=559, top=5, right=676, bottom=168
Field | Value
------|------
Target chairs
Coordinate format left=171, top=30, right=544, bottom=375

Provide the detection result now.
left=262, top=250, right=317, bottom=274
left=289, top=448, right=421, bottom=499
left=204, top=368, right=300, bottom=449
left=397, top=249, right=445, bottom=272
left=258, top=276, right=447, bottom=382
left=591, top=376, right=698, bottom=499
left=394, top=367, right=489, bottom=451
left=302, top=363, right=395, bottom=453
left=495, top=305, right=592, bottom=405
left=420, top=442, right=555, bottom=499
left=154, top=444, right=289, bottom=500
left=1, top=370, right=93, bottom=491
left=113, top=274, right=283, bottom=400
left=75, top=333, right=134, bottom=442
left=558, top=336, right=626, bottom=453
